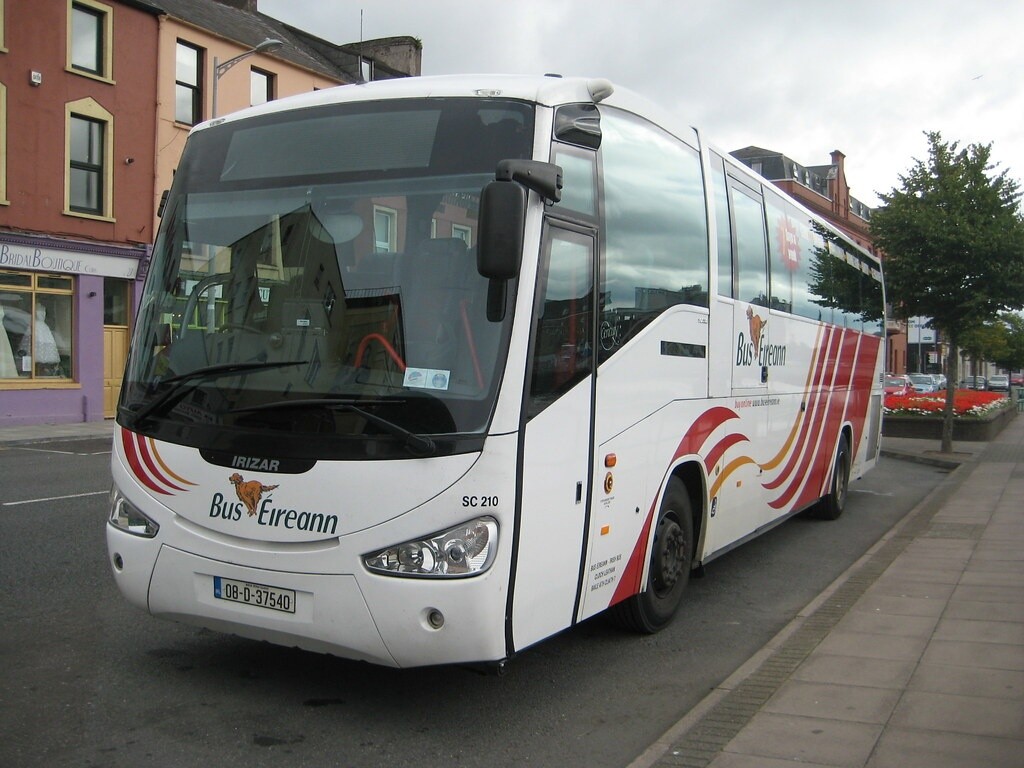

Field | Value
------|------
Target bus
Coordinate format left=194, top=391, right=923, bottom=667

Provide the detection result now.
left=104, top=77, right=888, bottom=672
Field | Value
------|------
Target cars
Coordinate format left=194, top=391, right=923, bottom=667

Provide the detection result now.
left=884, top=373, right=917, bottom=397
left=1010, top=373, right=1024, bottom=387
left=989, top=375, right=1010, bottom=392
left=909, top=373, right=939, bottom=395
left=929, top=373, right=947, bottom=391
left=962, top=376, right=989, bottom=391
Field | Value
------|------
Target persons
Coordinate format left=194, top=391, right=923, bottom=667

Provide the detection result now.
left=0, top=305, right=18, bottom=376
left=16, top=303, right=61, bottom=365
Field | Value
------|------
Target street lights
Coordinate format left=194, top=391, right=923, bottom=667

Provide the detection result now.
left=213, top=38, right=283, bottom=120
